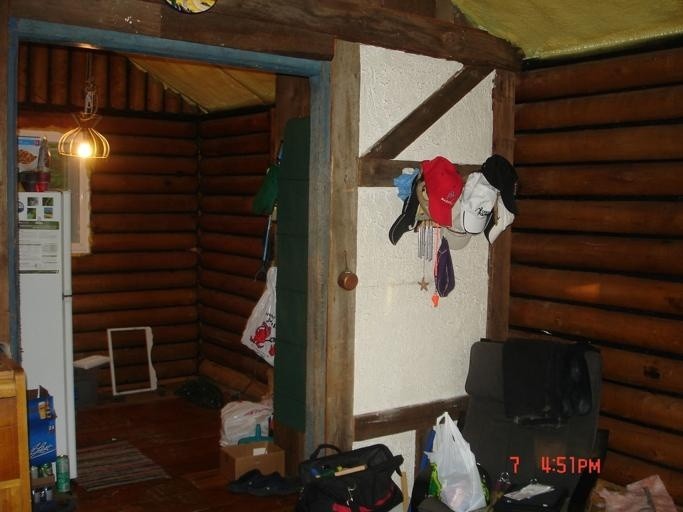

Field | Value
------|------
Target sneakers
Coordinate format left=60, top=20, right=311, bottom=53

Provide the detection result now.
left=226, top=469, right=299, bottom=497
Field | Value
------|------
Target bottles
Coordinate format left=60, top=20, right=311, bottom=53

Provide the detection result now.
left=37, top=138, right=52, bottom=184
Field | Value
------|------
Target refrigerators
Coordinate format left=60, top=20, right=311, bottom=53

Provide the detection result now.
left=15, top=186, right=79, bottom=481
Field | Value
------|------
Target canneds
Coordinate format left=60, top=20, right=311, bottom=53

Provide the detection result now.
left=32, top=455, right=72, bottom=504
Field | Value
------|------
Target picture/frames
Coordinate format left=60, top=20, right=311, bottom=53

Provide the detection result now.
left=18, top=127, right=91, bottom=256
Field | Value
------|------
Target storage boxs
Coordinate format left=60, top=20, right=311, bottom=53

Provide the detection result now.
left=220, top=442, right=285, bottom=481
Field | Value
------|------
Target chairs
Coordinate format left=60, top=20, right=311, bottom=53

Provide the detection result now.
left=407, top=338, right=610, bottom=512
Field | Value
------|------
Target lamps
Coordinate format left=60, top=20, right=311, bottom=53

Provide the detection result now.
left=58, top=53, right=110, bottom=159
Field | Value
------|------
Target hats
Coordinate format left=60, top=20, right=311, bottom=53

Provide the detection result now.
left=385, top=152, right=519, bottom=252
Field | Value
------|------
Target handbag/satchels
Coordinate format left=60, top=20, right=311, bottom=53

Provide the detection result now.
left=298, top=441, right=405, bottom=512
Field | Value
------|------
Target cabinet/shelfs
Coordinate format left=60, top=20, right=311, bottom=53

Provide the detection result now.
left=0, top=352, right=34, bottom=512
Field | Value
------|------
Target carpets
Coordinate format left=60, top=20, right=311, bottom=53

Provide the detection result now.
left=72, top=438, right=173, bottom=494
